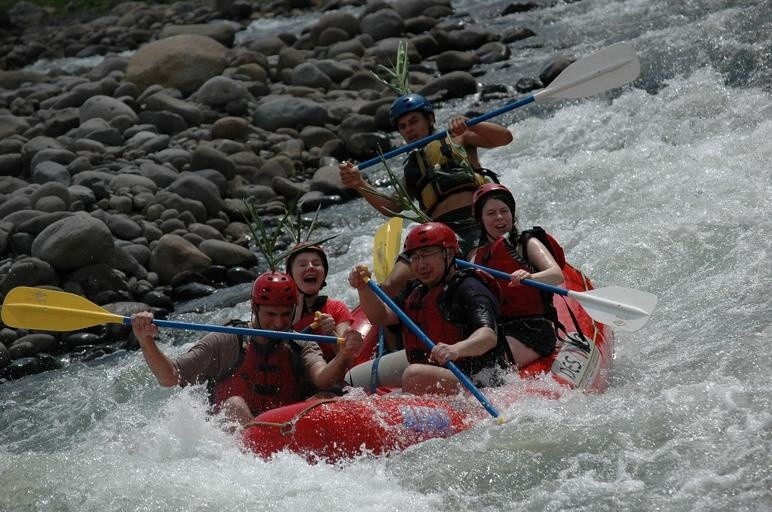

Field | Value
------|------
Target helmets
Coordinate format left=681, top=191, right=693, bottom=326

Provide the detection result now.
left=403, top=222, right=459, bottom=255
left=389, top=93, right=435, bottom=128
left=251, top=243, right=329, bottom=306
left=472, top=183, right=515, bottom=216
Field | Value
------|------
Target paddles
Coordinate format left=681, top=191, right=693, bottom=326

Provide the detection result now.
left=372, top=217, right=405, bottom=358
left=1, top=286, right=347, bottom=349
left=454, top=257, right=656, bottom=331
left=352, top=43, right=641, bottom=171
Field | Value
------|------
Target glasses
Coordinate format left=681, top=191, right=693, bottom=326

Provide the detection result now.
left=409, top=252, right=436, bottom=263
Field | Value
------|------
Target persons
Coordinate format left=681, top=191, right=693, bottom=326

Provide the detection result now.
left=344, top=222, right=517, bottom=393
left=470, top=183, right=567, bottom=373
left=285, top=244, right=355, bottom=362
left=339, top=94, right=514, bottom=296
left=131, top=270, right=363, bottom=426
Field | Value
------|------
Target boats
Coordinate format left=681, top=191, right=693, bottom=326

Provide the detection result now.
left=237, top=254, right=616, bottom=469
